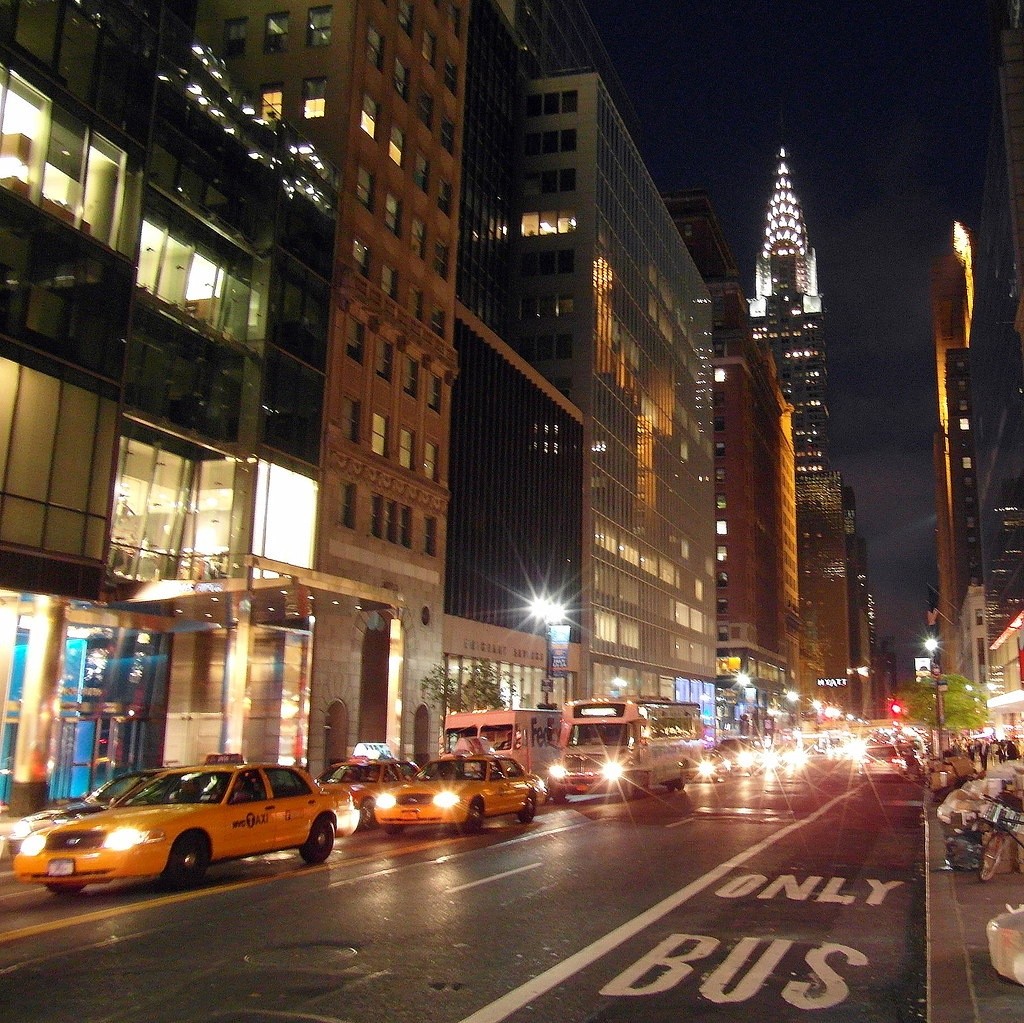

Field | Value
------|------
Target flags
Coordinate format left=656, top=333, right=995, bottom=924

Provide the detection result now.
left=925, top=605, right=939, bottom=626
left=927, top=584, right=940, bottom=613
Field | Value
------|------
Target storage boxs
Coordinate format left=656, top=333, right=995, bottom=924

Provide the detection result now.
left=930, top=755, right=972, bottom=789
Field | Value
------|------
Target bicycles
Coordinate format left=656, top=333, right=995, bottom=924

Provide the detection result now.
left=971, top=791, right=1024, bottom=883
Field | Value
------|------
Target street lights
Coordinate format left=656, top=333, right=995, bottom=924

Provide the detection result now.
left=529, top=598, right=567, bottom=710
left=925, top=636, right=947, bottom=763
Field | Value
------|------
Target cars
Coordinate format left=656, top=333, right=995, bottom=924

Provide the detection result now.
left=373, top=736, right=547, bottom=836
left=12, top=751, right=363, bottom=896
left=690, top=727, right=872, bottom=784
left=6, top=764, right=217, bottom=854
left=314, top=741, right=423, bottom=833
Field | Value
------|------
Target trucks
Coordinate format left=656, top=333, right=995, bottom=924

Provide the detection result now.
left=442, top=708, right=564, bottom=782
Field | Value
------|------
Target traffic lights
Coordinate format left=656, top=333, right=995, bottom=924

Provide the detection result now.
left=891, top=703, right=902, bottom=714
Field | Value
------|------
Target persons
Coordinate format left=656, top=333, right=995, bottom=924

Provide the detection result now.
left=951, top=736, right=1021, bottom=771
left=169, top=773, right=252, bottom=805
left=497, top=732, right=516, bottom=752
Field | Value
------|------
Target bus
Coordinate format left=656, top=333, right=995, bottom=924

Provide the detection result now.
left=545, top=695, right=705, bottom=803
